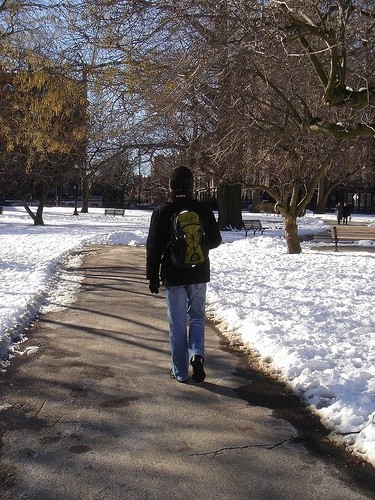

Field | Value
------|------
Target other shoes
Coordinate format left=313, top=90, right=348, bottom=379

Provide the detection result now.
left=191, top=356, right=206, bottom=383
left=169, top=367, right=190, bottom=382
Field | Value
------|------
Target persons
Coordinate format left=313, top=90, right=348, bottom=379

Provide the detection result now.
left=342, top=203, right=351, bottom=225
left=335, top=203, right=343, bottom=224
left=145, top=165, right=223, bottom=384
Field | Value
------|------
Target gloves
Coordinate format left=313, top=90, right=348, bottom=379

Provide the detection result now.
left=148, top=275, right=160, bottom=294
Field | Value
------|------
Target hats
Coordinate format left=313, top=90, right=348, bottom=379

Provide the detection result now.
left=170, top=166, right=194, bottom=193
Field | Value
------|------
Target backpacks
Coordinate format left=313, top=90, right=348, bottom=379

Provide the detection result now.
left=165, top=201, right=208, bottom=272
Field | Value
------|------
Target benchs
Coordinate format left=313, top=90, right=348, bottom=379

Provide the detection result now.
left=241, top=220, right=268, bottom=237
left=105, top=209, right=125, bottom=217
left=330, top=226, right=375, bottom=252
